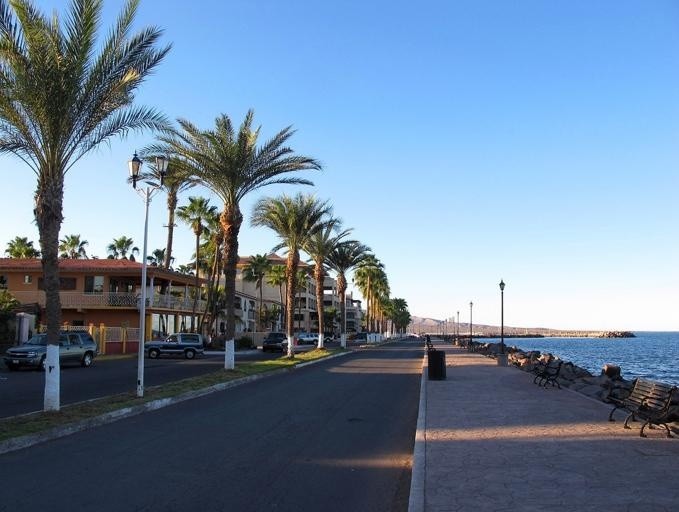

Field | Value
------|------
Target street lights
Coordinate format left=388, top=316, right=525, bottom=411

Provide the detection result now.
left=469, top=300, right=474, bottom=346
left=497, top=277, right=509, bottom=367
left=435, top=311, right=462, bottom=345
left=124, top=147, right=177, bottom=401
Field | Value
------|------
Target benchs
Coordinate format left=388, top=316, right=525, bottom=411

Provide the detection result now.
left=528, top=358, right=563, bottom=390
left=604, top=376, right=679, bottom=439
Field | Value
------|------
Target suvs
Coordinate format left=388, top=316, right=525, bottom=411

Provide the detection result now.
left=3, top=327, right=100, bottom=372
left=295, top=333, right=319, bottom=346
left=261, top=331, right=288, bottom=354
left=144, top=329, right=205, bottom=360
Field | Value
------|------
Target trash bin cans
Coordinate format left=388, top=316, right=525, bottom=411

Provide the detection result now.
left=428, top=351, right=446, bottom=380
